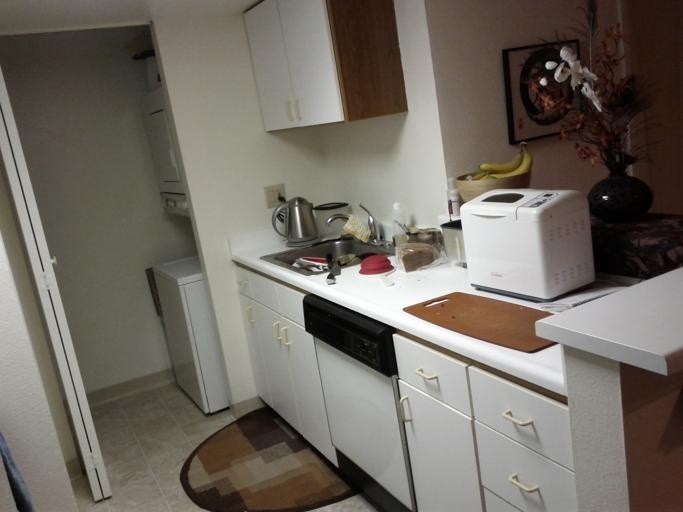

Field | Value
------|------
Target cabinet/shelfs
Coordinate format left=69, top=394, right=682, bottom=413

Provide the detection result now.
left=232, top=263, right=271, bottom=409
left=312, top=336, right=416, bottom=511
left=389, top=331, right=485, bottom=511
left=139, top=87, right=182, bottom=195
left=466, top=363, right=578, bottom=512
left=248, top=273, right=337, bottom=470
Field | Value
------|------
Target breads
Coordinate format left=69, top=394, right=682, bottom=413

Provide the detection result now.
left=394, top=244, right=436, bottom=273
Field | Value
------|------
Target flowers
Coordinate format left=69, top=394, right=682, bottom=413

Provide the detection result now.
left=533, top=46, right=660, bottom=174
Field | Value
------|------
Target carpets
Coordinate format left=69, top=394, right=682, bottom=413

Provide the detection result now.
left=180, top=404, right=360, bottom=510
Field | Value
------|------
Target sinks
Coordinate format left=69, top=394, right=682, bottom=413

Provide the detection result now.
left=258, top=235, right=396, bottom=276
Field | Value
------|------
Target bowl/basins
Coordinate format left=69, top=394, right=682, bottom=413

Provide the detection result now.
left=456, top=172, right=531, bottom=202
left=359, top=255, right=394, bottom=275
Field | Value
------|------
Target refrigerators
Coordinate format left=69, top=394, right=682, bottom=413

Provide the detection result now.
left=149, top=250, right=230, bottom=417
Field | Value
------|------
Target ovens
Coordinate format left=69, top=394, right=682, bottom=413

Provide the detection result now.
left=139, top=86, right=193, bottom=218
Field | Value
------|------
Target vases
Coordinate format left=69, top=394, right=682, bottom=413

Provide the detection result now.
left=585, top=155, right=652, bottom=223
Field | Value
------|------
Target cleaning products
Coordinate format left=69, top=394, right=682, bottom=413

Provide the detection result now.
left=391, top=202, right=409, bottom=248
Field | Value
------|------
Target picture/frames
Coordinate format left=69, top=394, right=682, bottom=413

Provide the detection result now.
left=502, top=41, right=584, bottom=146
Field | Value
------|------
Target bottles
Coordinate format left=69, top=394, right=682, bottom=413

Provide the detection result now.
left=392, top=202, right=409, bottom=267
left=446, top=178, right=460, bottom=215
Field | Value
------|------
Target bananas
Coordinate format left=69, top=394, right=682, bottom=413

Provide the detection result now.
left=471, top=142, right=532, bottom=180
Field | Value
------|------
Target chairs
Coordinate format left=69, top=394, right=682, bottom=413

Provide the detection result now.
left=241, top=0, right=408, bottom=133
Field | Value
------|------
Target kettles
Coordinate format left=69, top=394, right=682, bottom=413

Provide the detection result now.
left=272, top=195, right=321, bottom=248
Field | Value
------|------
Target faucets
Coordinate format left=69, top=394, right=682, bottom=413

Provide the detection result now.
left=325, top=203, right=380, bottom=241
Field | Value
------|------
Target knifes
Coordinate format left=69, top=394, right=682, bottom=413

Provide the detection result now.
left=326, top=264, right=341, bottom=285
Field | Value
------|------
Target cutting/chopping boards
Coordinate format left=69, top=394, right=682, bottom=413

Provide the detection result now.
left=403, top=290, right=560, bottom=354
